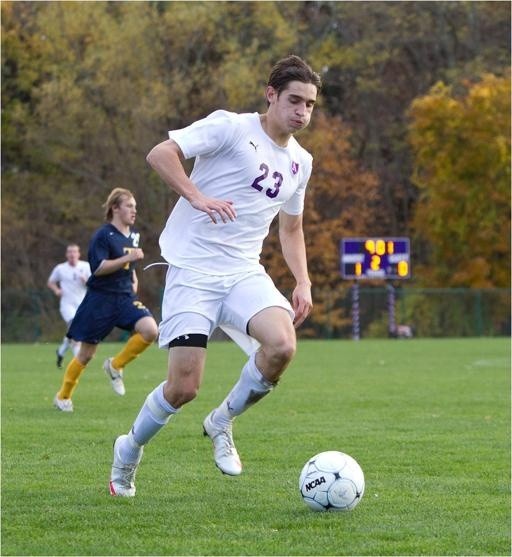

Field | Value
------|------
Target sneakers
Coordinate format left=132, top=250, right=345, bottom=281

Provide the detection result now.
left=101, top=355, right=127, bottom=396
left=52, top=391, right=74, bottom=413
left=201, top=408, right=241, bottom=477
left=55, top=348, right=64, bottom=368
left=108, top=432, right=144, bottom=499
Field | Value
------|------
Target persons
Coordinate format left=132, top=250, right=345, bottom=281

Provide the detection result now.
left=54, top=188, right=157, bottom=410
left=46, top=241, right=92, bottom=367
left=110, top=51, right=324, bottom=496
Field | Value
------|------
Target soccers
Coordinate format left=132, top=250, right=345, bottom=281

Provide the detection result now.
left=298, top=450, right=365, bottom=513
